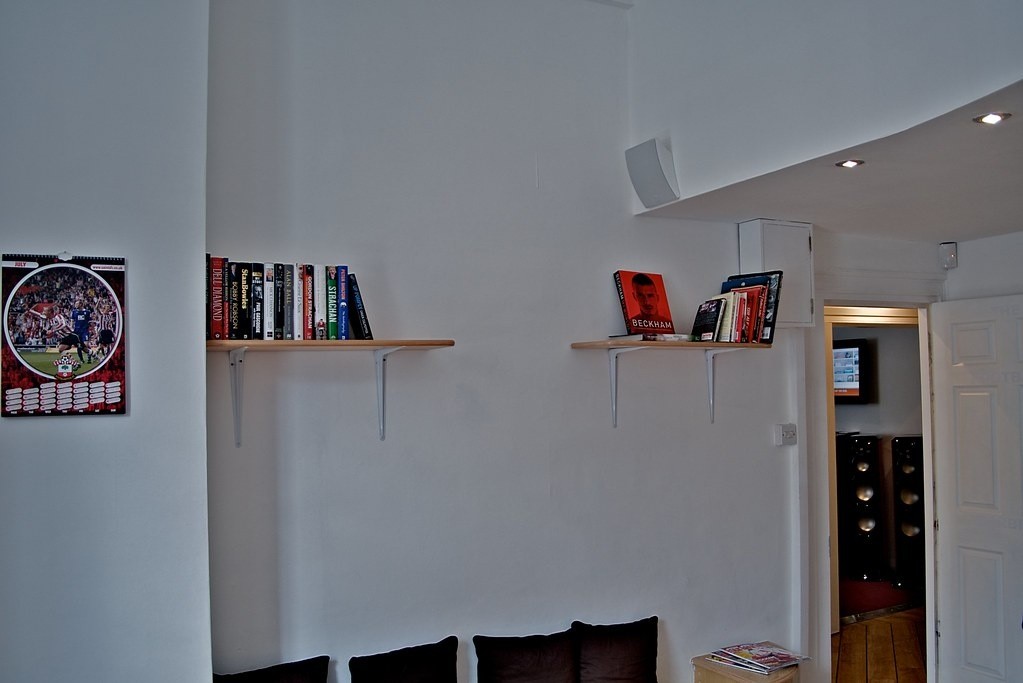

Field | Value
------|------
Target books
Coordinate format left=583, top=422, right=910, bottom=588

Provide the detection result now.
left=705, top=643, right=812, bottom=674
left=608, top=270, right=694, bottom=341
left=691, top=269, right=783, bottom=344
left=206, top=252, right=373, bottom=340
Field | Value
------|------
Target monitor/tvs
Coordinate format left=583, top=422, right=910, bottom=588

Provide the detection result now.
left=833, top=338, right=873, bottom=404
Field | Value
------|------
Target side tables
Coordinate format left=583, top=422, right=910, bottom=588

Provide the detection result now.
left=690, top=654, right=799, bottom=682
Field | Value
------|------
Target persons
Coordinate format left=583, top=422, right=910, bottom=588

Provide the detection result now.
left=629, top=273, right=672, bottom=330
left=8, top=266, right=116, bottom=364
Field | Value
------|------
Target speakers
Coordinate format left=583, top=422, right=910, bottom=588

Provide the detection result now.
left=890, top=434, right=926, bottom=587
left=623, top=138, right=680, bottom=209
left=837, top=433, right=889, bottom=581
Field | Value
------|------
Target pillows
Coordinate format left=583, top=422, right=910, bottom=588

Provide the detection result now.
left=472, top=628, right=571, bottom=682
left=213, top=655, right=330, bottom=682
left=348, top=636, right=458, bottom=683
left=571, top=616, right=658, bottom=683
